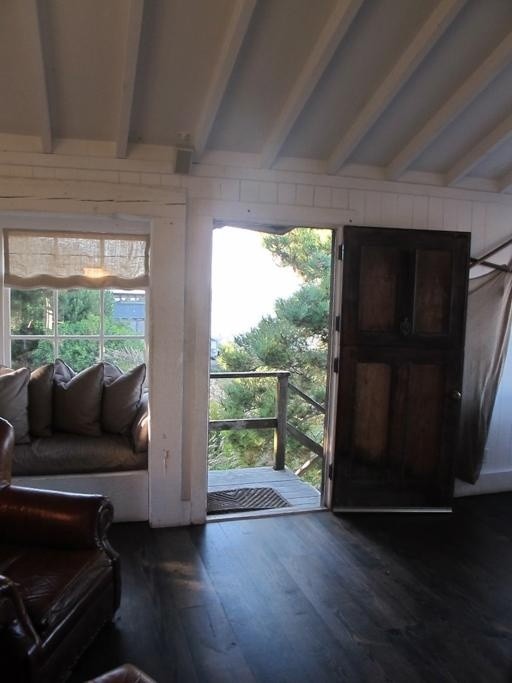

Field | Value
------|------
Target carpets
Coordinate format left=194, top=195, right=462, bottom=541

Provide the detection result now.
left=204, top=486, right=290, bottom=515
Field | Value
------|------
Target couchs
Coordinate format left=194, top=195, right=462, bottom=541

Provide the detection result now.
left=0, top=398, right=149, bottom=526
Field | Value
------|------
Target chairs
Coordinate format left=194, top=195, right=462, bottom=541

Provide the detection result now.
left=0, top=417, right=122, bottom=683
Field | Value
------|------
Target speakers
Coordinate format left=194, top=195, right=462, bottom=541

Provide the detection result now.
left=175, top=142, right=194, bottom=175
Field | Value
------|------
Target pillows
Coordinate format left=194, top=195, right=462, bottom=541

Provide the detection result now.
left=0, top=357, right=147, bottom=442
